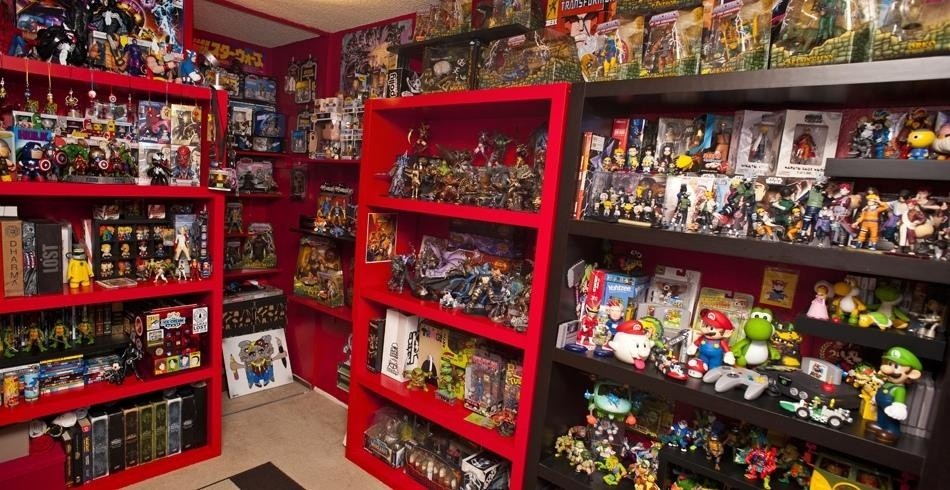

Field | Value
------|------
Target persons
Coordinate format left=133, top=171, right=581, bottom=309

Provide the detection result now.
left=208, top=67, right=362, bottom=298
left=1, top=1, right=201, bottom=286
left=367, top=1, right=950, bottom=489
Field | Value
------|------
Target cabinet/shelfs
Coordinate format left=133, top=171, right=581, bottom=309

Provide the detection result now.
left=0, top=1, right=950, bottom=490
